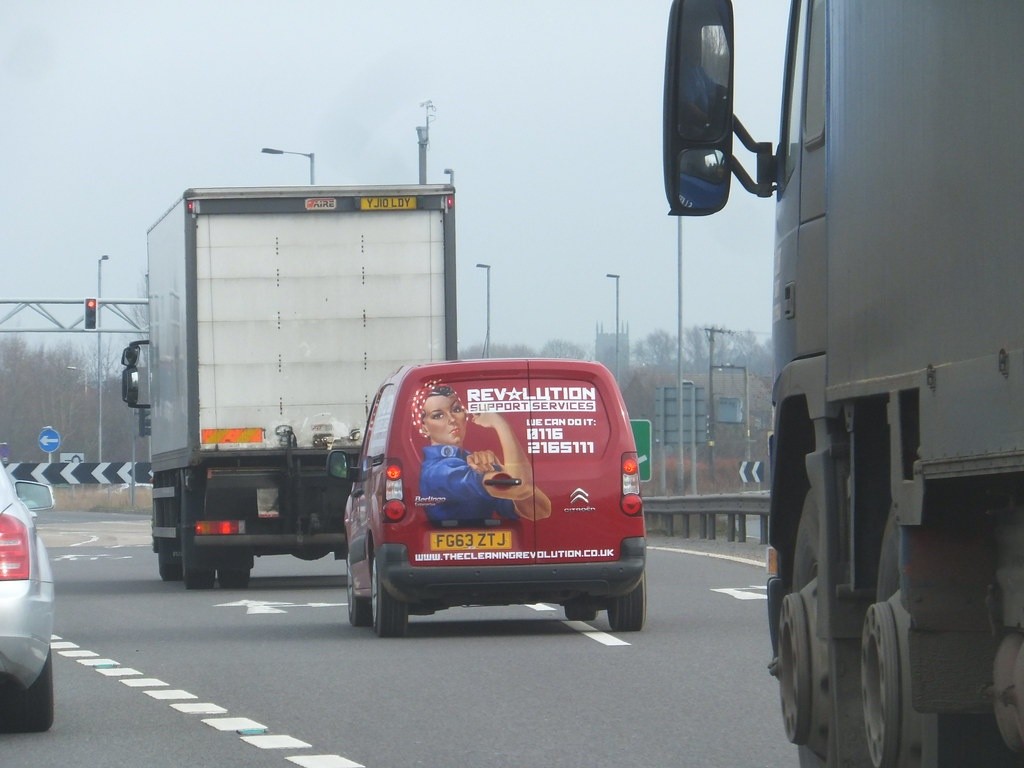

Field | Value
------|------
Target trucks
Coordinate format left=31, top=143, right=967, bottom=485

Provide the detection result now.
left=121, top=184, right=459, bottom=590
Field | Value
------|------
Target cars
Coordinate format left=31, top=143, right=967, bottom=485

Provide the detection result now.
left=0, top=460, right=58, bottom=731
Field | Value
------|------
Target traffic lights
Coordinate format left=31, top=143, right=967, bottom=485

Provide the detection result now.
left=84, top=298, right=97, bottom=329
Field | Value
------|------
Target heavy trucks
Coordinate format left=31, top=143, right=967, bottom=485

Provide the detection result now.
left=662, top=0, right=1024, bottom=767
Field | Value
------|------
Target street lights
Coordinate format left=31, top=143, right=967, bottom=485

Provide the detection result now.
left=474, top=262, right=491, bottom=358
left=606, top=274, right=620, bottom=382
left=95, top=255, right=110, bottom=463
left=259, top=146, right=316, bottom=185
left=443, top=169, right=455, bottom=183
left=68, top=366, right=89, bottom=401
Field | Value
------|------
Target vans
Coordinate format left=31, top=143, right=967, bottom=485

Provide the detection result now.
left=326, top=355, right=647, bottom=638
